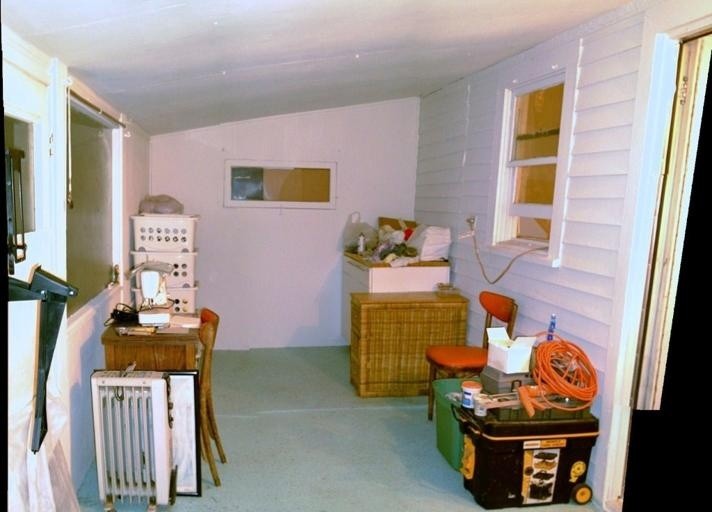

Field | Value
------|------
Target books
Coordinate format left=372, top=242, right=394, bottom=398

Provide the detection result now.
left=127, top=315, right=201, bottom=337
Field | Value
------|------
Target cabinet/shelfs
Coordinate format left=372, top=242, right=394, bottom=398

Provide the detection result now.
left=341, top=254, right=450, bottom=347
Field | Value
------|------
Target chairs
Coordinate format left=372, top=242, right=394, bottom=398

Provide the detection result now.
left=196, top=322, right=226, bottom=486
left=425, top=290, right=519, bottom=420
left=198, top=308, right=219, bottom=459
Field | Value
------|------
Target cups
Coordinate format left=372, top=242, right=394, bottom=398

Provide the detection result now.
left=461, top=380, right=484, bottom=409
left=472, top=394, right=489, bottom=417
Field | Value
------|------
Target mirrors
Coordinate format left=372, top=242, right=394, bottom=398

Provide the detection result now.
left=3, top=110, right=36, bottom=235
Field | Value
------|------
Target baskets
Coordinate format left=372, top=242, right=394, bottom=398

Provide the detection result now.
left=129, top=215, right=197, bottom=253
left=130, top=250, right=197, bottom=288
left=131, top=287, right=197, bottom=315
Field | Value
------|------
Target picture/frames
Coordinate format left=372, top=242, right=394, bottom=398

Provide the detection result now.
left=224, top=159, right=337, bottom=210
left=93, top=370, right=202, bottom=498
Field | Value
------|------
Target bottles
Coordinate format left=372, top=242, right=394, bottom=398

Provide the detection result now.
left=357, top=232, right=366, bottom=255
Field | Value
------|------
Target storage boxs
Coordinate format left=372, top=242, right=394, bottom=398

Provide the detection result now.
left=430, top=375, right=487, bottom=473
left=485, top=327, right=537, bottom=375
left=451, top=404, right=600, bottom=511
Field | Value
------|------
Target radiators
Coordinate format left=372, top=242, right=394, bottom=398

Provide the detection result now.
left=90, top=368, right=178, bottom=512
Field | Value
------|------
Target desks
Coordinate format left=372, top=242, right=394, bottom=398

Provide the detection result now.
left=348, top=292, right=469, bottom=397
left=100, top=304, right=201, bottom=372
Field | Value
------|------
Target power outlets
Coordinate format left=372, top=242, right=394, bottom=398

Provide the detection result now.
left=465, top=212, right=477, bottom=228
